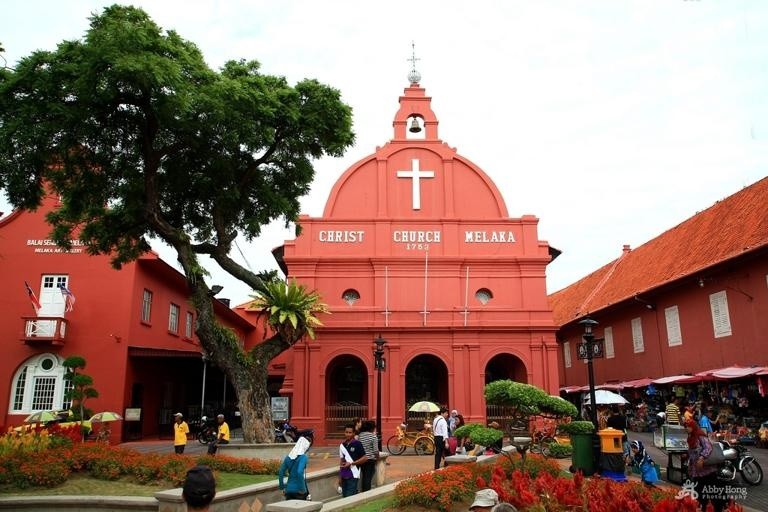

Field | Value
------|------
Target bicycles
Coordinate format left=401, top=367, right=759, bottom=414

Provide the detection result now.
left=387, top=422, right=436, bottom=455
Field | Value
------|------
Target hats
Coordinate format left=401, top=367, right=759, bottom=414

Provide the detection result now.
left=183, top=466, right=215, bottom=498
left=173, top=413, right=183, bottom=418
left=488, top=422, right=499, bottom=429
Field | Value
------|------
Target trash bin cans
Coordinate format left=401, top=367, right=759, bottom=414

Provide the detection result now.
left=595, top=426, right=629, bottom=482
left=569, top=432, right=595, bottom=477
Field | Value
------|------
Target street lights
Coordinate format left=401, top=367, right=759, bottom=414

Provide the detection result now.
left=577, top=310, right=599, bottom=440
left=375, top=334, right=386, bottom=439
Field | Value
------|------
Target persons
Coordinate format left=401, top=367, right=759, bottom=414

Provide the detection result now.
left=684, top=417, right=728, bottom=511
left=357, top=420, right=380, bottom=493
left=207, top=413, right=231, bottom=454
left=172, top=410, right=190, bottom=457
left=625, top=439, right=660, bottom=485
left=574, top=388, right=750, bottom=434
left=276, top=432, right=311, bottom=500
left=180, top=464, right=218, bottom=512
left=432, top=406, right=503, bottom=468
left=335, top=424, right=369, bottom=497
left=351, top=415, right=363, bottom=442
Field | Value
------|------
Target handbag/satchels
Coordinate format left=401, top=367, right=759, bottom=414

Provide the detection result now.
left=704, top=443, right=725, bottom=466
left=465, top=442, right=475, bottom=451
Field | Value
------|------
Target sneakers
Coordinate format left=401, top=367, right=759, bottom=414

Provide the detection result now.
left=435, top=463, right=448, bottom=469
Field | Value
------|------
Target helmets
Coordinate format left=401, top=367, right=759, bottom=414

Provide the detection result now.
left=201, top=416, right=208, bottom=421
left=281, top=418, right=289, bottom=424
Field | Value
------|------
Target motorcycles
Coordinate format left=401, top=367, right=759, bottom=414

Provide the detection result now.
left=275, top=418, right=316, bottom=446
left=197, top=420, right=218, bottom=444
left=717, top=435, right=763, bottom=488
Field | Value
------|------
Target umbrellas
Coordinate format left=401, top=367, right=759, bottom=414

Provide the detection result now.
left=88, top=406, right=125, bottom=425
left=408, top=400, right=441, bottom=423
left=22, top=408, right=64, bottom=424
